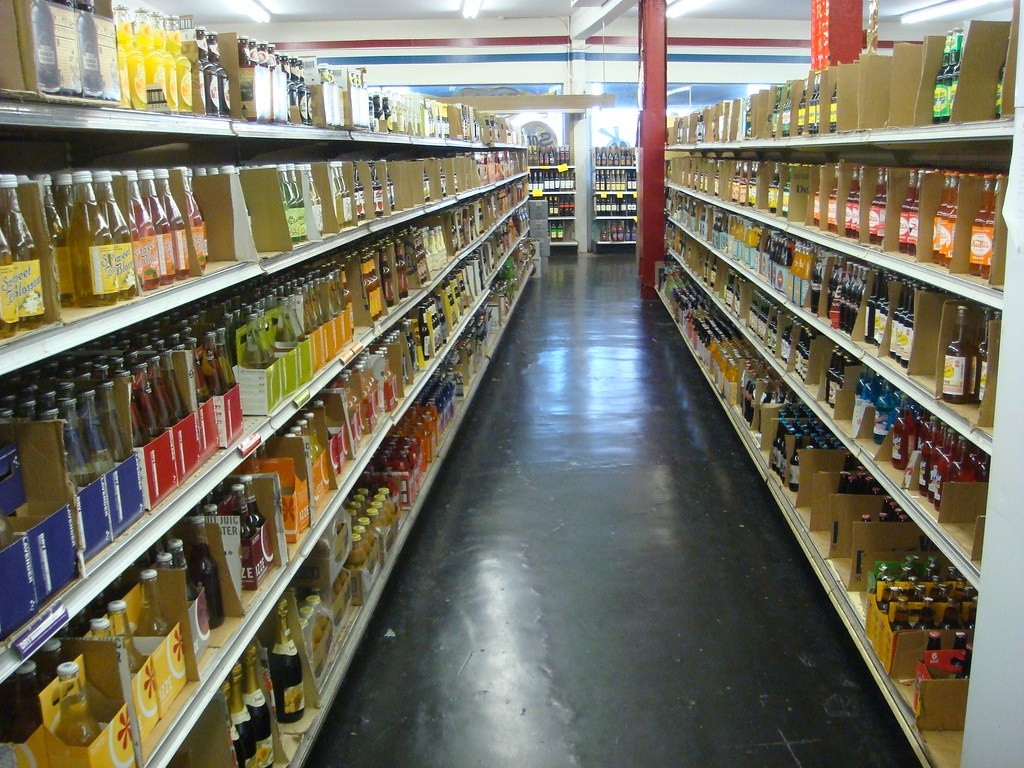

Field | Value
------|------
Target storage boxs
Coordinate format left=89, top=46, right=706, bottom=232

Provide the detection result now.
left=863, top=559, right=978, bottom=732
left=531, top=237, right=551, bottom=256
left=0, top=0, right=528, bottom=146
left=0, top=308, right=506, bottom=768
left=530, top=220, right=549, bottom=238
left=530, top=257, right=542, bottom=279
left=528, top=199, right=549, bottom=220
left=530, top=241, right=541, bottom=260
left=541, top=257, right=549, bottom=273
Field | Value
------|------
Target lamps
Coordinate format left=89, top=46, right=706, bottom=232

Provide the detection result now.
left=461, top=0, right=482, bottom=19
left=899, top=0, right=1024, bottom=24
left=666, top=0, right=714, bottom=20
left=228, top=0, right=272, bottom=24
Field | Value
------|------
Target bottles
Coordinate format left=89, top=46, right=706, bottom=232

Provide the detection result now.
left=270, top=599, right=305, bottom=724
left=9, top=660, right=44, bottom=743
left=528, top=145, right=574, bottom=166
left=0, top=674, right=15, bottom=743
left=228, top=661, right=259, bottom=768
left=529, top=169, right=576, bottom=191
left=658, top=28, right=1016, bottom=725
left=218, top=681, right=245, bottom=768
left=529, top=194, right=638, bottom=242
left=55, top=662, right=102, bottom=747
left=597, top=147, right=637, bottom=166
left=245, top=644, right=274, bottom=768
left=0, top=1, right=529, bottom=688
left=596, top=170, right=638, bottom=192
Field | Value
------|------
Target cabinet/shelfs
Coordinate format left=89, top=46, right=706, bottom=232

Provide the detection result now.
left=0, top=97, right=538, bottom=768
left=529, top=165, right=579, bottom=247
left=595, top=165, right=637, bottom=245
left=652, top=118, right=1024, bottom=768
